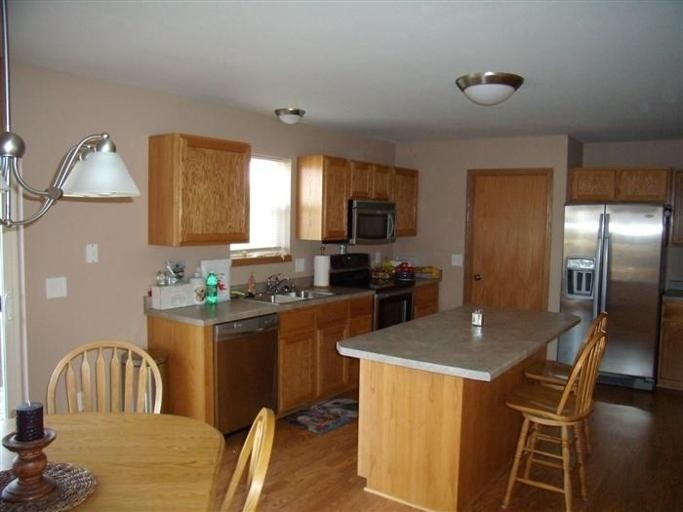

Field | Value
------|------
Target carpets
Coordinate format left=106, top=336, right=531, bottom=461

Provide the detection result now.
left=284, top=398, right=359, bottom=434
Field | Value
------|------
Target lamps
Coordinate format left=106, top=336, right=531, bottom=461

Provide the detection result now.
left=455, top=72, right=525, bottom=106
left=274, top=108, right=306, bottom=125
left=0, top=132, right=140, bottom=228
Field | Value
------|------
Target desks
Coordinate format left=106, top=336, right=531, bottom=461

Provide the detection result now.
left=1, top=412, right=225, bottom=512
left=336, top=305, right=581, bottom=512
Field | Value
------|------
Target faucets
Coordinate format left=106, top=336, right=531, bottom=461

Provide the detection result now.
left=264, top=271, right=292, bottom=293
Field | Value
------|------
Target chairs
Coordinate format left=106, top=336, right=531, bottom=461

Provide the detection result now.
left=500, top=312, right=607, bottom=512
left=220, top=406, right=275, bottom=512
left=46, top=340, right=164, bottom=414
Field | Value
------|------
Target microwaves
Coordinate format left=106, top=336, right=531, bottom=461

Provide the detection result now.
left=348, top=199, right=397, bottom=244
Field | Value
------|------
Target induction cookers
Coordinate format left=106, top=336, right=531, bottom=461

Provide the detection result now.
left=329, top=276, right=415, bottom=295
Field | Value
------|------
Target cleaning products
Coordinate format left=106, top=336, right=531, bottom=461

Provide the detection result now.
left=246, top=270, right=257, bottom=295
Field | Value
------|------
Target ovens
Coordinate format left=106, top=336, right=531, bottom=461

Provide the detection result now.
left=372, top=291, right=414, bottom=331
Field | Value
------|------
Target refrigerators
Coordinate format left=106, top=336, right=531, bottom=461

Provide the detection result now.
left=555, top=203, right=668, bottom=393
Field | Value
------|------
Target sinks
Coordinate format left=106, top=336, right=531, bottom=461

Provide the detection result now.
left=276, top=289, right=338, bottom=300
left=240, top=291, right=307, bottom=306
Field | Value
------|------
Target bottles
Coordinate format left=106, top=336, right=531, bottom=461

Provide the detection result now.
left=471, top=309, right=487, bottom=327
left=190, top=267, right=218, bottom=306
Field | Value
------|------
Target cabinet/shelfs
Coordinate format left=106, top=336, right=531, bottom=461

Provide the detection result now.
left=392, top=166, right=418, bottom=237
left=567, top=167, right=671, bottom=204
left=656, top=299, right=683, bottom=391
left=149, top=133, right=252, bottom=248
left=277, top=294, right=372, bottom=421
left=296, top=155, right=349, bottom=241
left=349, top=161, right=391, bottom=200
left=412, top=282, right=438, bottom=319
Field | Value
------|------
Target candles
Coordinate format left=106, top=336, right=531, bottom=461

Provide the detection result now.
left=16, top=398, right=43, bottom=442
